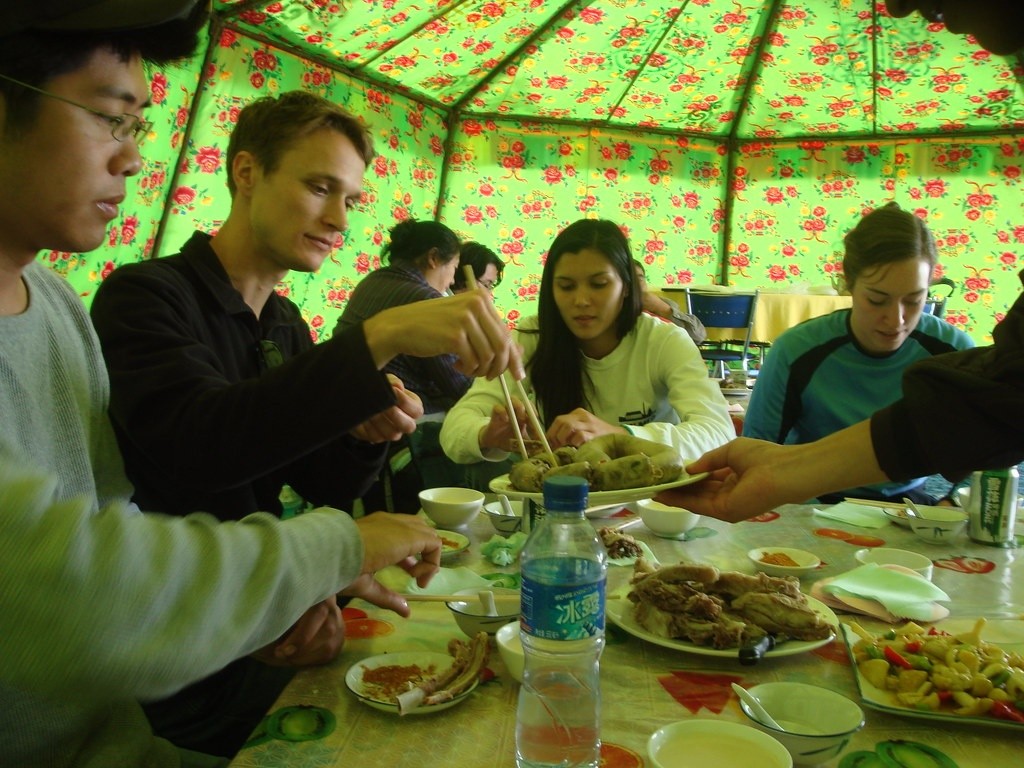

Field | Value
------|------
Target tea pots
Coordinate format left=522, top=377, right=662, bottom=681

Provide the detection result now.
left=829, top=274, right=852, bottom=296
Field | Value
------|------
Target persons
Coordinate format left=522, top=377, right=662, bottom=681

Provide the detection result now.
left=0, top=0, right=442, bottom=768
left=438, top=218, right=738, bottom=467
left=449, top=241, right=505, bottom=295
left=89, top=89, right=525, bottom=524
left=633, top=259, right=707, bottom=346
left=652, top=267, right=1024, bottom=523
left=333, top=217, right=513, bottom=516
left=741, top=202, right=1024, bottom=505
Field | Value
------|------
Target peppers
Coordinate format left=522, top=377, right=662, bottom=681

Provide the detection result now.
left=987, top=698, right=1024, bottom=722
left=927, top=627, right=939, bottom=635
left=906, top=642, right=923, bottom=654
left=938, top=689, right=951, bottom=703
left=884, top=645, right=911, bottom=668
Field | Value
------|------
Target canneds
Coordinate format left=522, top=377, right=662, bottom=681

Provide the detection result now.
left=968, top=464, right=1019, bottom=546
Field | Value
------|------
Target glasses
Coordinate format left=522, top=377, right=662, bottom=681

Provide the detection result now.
left=476, top=277, right=495, bottom=290
left=0, top=73, right=155, bottom=147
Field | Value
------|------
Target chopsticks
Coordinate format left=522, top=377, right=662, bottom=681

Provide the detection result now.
left=398, top=593, right=521, bottom=603
left=844, top=498, right=928, bottom=509
left=462, top=264, right=560, bottom=470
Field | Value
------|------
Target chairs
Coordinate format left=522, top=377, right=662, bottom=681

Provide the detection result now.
left=685, top=288, right=759, bottom=379
left=922, top=278, right=956, bottom=319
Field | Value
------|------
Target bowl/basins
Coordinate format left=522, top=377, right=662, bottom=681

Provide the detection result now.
left=496, top=621, right=606, bottom=685
left=418, top=488, right=485, bottom=530
left=957, top=487, right=1023, bottom=518
left=739, top=682, right=866, bottom=767
left=647, top=719, right=794, bottom=768
left=445, top=587, right=521, bottom=644
left=484, top=501, right=524, bottom=539
left=904, top=506, right=970, bottom=545
left=636, top=499, right=700, bottom=538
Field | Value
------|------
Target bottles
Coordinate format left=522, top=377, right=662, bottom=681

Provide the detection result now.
left=514, top=475, right=609, bottom=768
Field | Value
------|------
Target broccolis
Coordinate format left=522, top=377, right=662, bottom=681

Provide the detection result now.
left=849, top=620, right=1024, bottom=717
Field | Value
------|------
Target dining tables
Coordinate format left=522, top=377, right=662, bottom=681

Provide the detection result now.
left=651, top=288, right=853, bottom=348
left=222, top=502, right=1024, bottom=768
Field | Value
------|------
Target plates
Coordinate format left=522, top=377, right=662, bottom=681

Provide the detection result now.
left=746, top=547, right=821, bottom=578
left=839, top=622, right=1024, bottom=730
left=412, top=530, right=471, bottom=563
left=721, top=388, right=753, bottom=395
left=605, top=583, right=840, bottom=658
left=344, top=651, right=479, bottom=713
left=883, top=508, right=911, bottom=529
left=489, top=457, right=709, bottom=507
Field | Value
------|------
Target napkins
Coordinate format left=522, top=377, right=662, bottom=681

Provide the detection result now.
left=822, top=561, right=952, bottom=625
left=407, top=565, right=505, bottom=597
left=812, top=502, right=899, bottom=528
